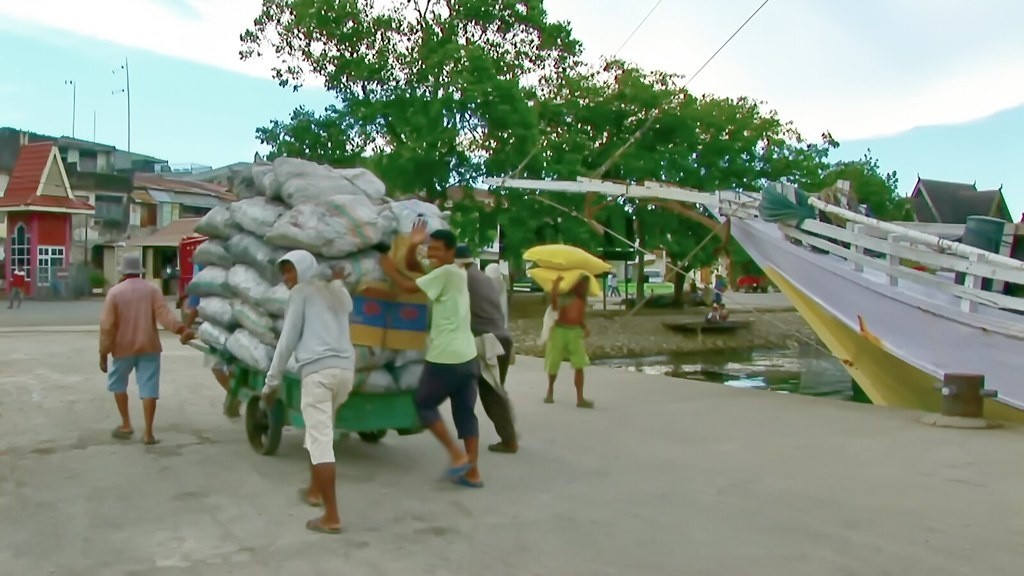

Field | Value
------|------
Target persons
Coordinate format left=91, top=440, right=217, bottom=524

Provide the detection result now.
left=542, top=272, right=595, bottom=408
left=98, top=253, right=195, bottom=445
left=7, top=263, right=26, bottom=309
left=377, top=218, right=519, bottom=489
left=259, top=249, right=355, bottom=534
left=690, top=278, right=698, bottom=297
left=606, top=271, right=622, bottom=297
left=708, top=274, right=729, bottom=324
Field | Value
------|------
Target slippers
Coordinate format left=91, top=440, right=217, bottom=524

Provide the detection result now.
left=114, top=428, right=130, bottom=440
left=307, top=518, right=341, bottom=533
left=141, top=434, right=154, bottom=444
left=446, top=464, right=471, bottom=476
left=456, top=475, right=483, bottom=487
left=300, top=487, right=323, bottom=506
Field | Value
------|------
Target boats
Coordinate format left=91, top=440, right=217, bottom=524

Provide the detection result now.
left=484, top=0, right=1024, bottom=430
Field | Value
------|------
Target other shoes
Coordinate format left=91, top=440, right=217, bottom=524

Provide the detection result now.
left=576, top=400, right=593, bottom=408
left=544, top=398, right=553, bottom=403
left=489, top=443, right=516, bottom=453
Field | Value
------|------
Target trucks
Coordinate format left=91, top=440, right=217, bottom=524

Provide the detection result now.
left=174, top=234, right=209, bottom=324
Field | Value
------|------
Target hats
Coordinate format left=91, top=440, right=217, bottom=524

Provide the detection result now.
left=453, top=243, right=474, bottom=263
left=116, top=253, right=146, bottom=274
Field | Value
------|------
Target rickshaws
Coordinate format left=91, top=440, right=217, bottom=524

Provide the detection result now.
left=179, top=338, right=429, bottom=456
